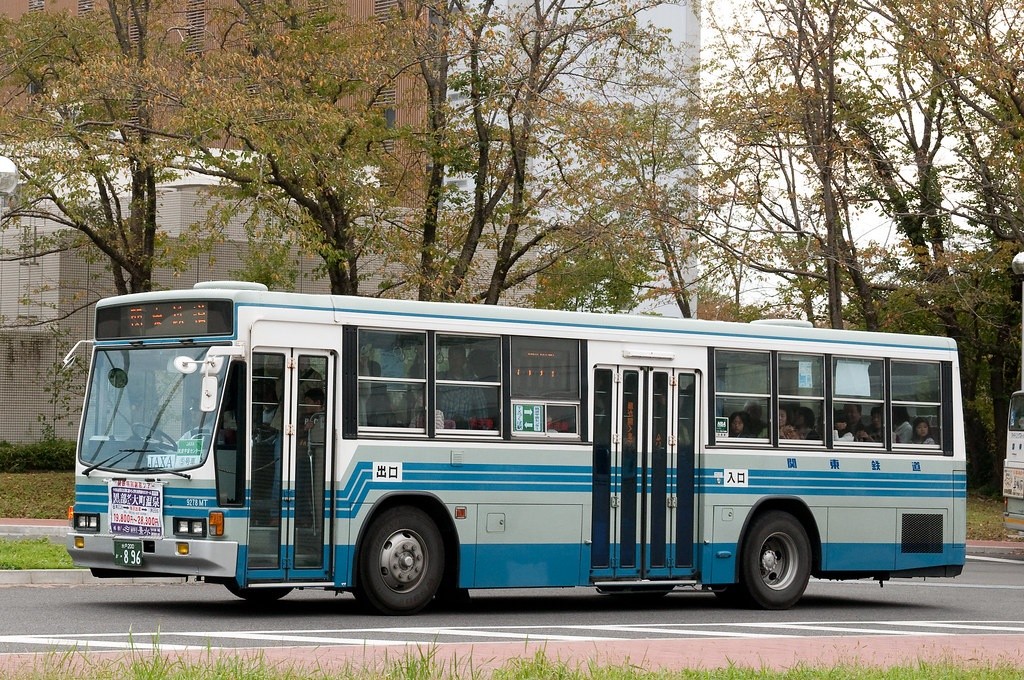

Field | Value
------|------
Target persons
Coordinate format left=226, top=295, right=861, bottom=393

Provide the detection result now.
left=892, top=399, right=935, bottom=444
left=729, top=402, right=768, bottom=438
left=266, top=357, right=326, bottom=526
left=170, top=399, right=229, bottom=453
left=779, top=407, right=820, bottom=440
left=406, top=345, right=489, bottom=429
left=359, top=356, right=397, bottom=426
left=833, top=398, right=883, bottom=443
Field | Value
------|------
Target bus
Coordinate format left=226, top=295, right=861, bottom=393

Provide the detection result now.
left=61, top=280, right=970, bottom=616
left=1002, top=391, right=1024, bottom=532
